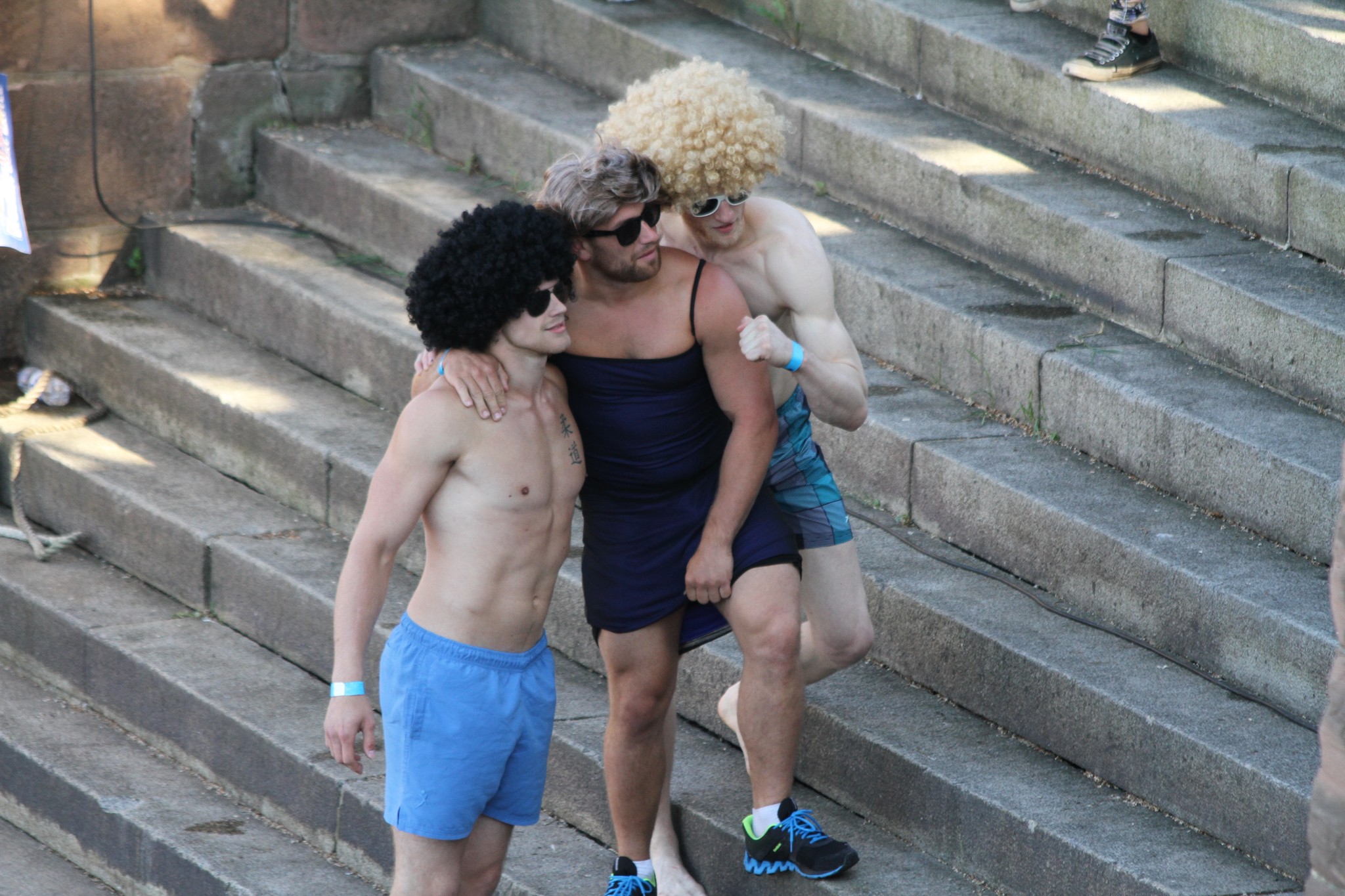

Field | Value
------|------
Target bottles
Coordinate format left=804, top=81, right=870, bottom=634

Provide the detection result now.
left=13, top=364, right=70, bottom=407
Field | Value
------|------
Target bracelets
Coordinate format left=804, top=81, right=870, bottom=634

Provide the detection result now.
left=438, top=348, right=451, bottom=375
left=330, top=681, right=365, bottom=697
left=782, top=340, right=804, bottom=373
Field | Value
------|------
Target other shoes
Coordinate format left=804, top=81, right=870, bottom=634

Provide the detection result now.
left=1010, top=0, right=1052, bottom=13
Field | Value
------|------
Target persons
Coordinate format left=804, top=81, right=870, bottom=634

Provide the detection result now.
left=1010, top=0, right=1163, bottom=82
left=322, top=53, right=875, bottom=896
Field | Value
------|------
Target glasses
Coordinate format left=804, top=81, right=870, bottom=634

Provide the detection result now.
left=527, top=278, right=569, bottom=317
left=689, top=186, right=751, bottom=217
left=588, top=198, right=661, bottom=246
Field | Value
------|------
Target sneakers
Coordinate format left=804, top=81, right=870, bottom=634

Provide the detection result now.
left=603, top=856, right=657, bottom=896
left=741, top=796, right=859, bottom=879
left=1062, top=18, right=1163, bottom=82
